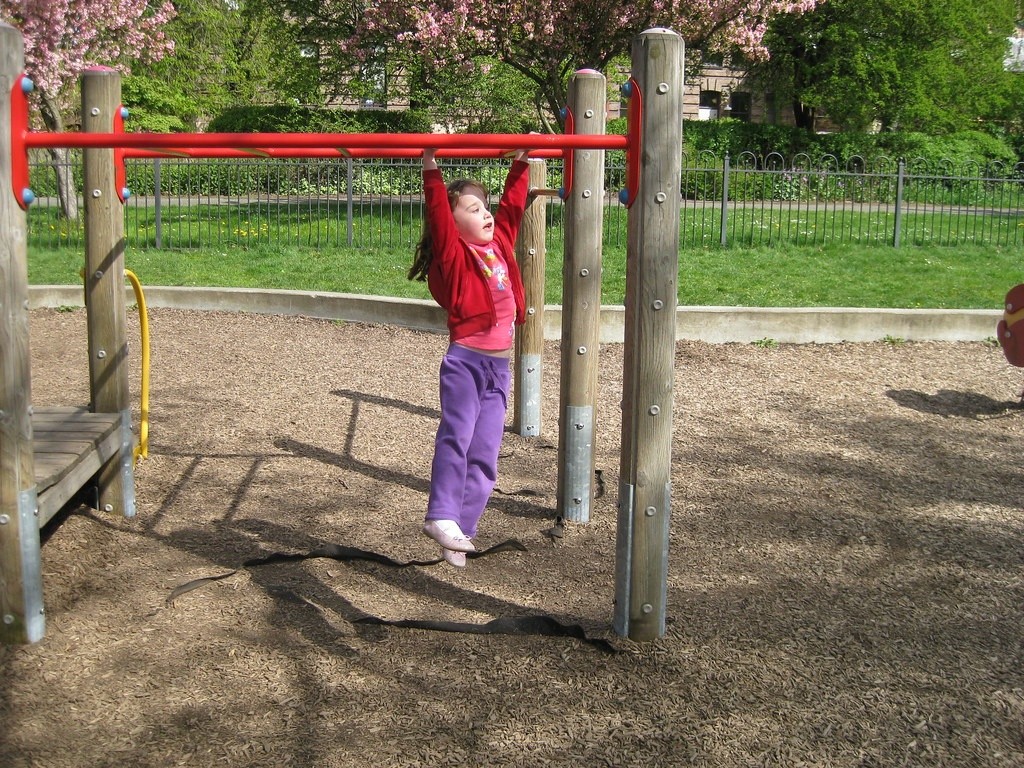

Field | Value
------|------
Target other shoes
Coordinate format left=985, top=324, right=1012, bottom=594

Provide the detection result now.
left=422, top=518, right=475, bottom=552
left=443, top=547, right=466, bottom=568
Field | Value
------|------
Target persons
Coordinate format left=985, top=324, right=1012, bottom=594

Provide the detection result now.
left=408, top=131, right=541, bottom=571
